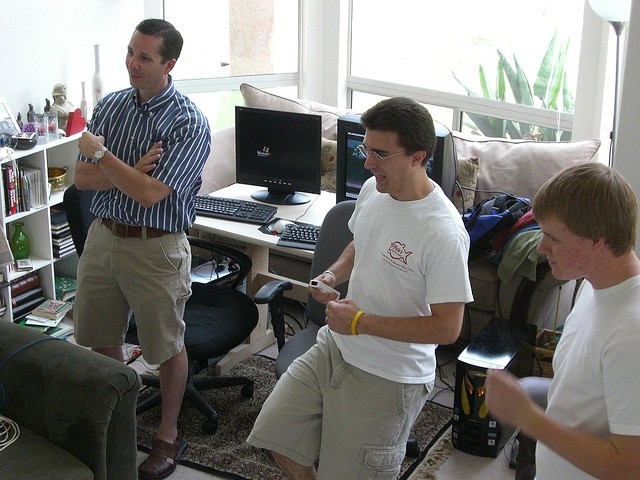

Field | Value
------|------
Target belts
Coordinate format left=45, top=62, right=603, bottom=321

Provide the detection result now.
left=102, top=218, right=165, bottom=238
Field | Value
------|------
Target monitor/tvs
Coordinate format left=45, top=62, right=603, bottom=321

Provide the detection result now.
left=335, top=108, right=460, bottom=205
left=235, top=106, right=322, bottom=205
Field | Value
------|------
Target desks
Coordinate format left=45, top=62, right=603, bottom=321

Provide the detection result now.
left=183, top=180, right=337, bottom=376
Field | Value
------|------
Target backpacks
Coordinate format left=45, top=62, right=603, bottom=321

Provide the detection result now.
left=462, top=194, right=531, bottom=260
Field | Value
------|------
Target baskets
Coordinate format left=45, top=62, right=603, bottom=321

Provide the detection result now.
left=532, top=328, right=562, bottom=378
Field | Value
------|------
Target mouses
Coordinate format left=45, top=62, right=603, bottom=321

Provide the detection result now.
left=267, top=218, right=300, bottom=234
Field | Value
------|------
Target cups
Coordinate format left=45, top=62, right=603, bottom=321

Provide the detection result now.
left=44, top=112, right=58, bottom=141
left=34, top=115, right=48, bottom=144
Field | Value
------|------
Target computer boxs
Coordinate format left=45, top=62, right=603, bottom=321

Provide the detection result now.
left=451, top=316, right=538, bottom=460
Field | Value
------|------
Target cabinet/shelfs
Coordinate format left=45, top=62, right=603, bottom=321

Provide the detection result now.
left=0, top=128, right=88, bottom=342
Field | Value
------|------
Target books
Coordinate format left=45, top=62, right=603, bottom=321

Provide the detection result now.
left=11, top=270, right=77, bottom=339
left=16, top=258, right=33, bottom=272
left=50, top=207, right=76, bottom=258
left=2, top=163, right=45, bottom=217
left=0, top=273, right=7, bottom=318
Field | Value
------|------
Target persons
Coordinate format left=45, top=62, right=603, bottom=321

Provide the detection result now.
left=16, top=112, right=23, bottom=129
left=52, top=82, right=73, bottom=130
left=74, top=19, right=211, bottom=479
left=27, top=104, right=34, bottom=122
left=245, top=98, right=475, bottom=480
left=44, top=98, right=50, bottom=112
left=485, top=162, right=640, bottom=480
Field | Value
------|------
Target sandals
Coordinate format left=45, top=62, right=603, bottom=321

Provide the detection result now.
left=138, top=435, right=187, bottom=479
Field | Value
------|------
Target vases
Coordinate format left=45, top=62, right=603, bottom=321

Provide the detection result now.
left=9, top=221, right=31, bottom=258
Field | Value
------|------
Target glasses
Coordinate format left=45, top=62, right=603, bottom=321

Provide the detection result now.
left=357, top=145, right=406, bottom=163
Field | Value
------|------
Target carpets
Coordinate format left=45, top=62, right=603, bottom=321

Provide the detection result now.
left=137, top=354, right=454, bottom=480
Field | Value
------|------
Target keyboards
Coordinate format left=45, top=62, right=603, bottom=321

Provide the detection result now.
left=194, top=194, right=279, bottom=224
left=276, top=223, right=322, bottom=251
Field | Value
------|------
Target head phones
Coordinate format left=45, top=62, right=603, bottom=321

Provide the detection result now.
left=214, top=236, right=240, bottom=276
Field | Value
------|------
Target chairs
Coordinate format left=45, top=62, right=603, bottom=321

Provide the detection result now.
left=255, top=199, right=421, bottom=463
left=63, top=182, right=259, bottom=434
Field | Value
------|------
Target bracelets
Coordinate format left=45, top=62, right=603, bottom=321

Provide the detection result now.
left=351, top=311, right=365, bottom=336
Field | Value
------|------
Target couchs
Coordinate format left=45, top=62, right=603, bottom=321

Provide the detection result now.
left=191, top=121, right=583, bottom=335
left=0, top=318, right=143, bottom=480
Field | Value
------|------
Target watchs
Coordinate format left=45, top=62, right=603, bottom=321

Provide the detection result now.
left=93, top=145, right=108, bottom=164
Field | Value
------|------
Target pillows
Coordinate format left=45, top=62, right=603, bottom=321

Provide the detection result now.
left=320, top=136, right=336, bottom=193
left=240, top=81, right=366, bottom=142
left=452, top=155, right=480, bottom=212
left=453, top=129, right=601, bottom=206
